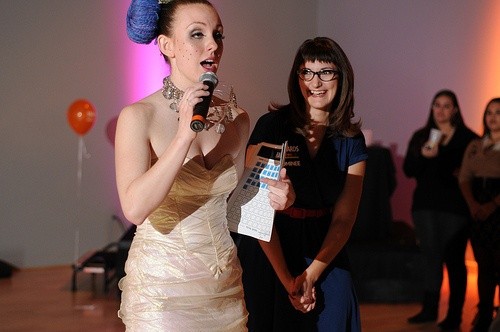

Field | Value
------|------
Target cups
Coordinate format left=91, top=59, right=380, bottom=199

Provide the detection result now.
left=427, top=129, right=443, bottom=149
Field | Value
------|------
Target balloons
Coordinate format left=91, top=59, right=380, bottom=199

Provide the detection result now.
left=67, top=98, right=96, bottom=137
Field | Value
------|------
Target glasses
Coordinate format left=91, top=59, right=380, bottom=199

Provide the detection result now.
left=296, top=67, right=341, bottom=82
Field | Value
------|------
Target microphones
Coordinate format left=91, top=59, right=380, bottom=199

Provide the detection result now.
left=190, top=71, right=218, bottom=132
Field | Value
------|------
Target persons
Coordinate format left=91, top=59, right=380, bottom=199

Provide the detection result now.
left=231, top=36, right=366, bottom=332
left=402, top=89, right=482, bottom=328
left=459, top=96, right=500, bottom=332
left=115, top=0, right=295, bottom=332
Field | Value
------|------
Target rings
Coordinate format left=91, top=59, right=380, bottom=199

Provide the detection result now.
left=186, top=99, right=188, bottom=101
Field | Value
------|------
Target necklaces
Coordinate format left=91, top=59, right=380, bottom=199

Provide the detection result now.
left=162, top=75, right=238, bottom=134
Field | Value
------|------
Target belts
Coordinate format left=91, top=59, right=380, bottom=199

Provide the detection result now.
left=274, top=206, right=331, bottom=220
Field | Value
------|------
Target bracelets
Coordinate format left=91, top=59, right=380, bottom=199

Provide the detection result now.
left=492, top=200, right=499, bottom=208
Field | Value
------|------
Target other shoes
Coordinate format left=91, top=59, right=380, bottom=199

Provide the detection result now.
left=468, top=313, right=495, bottom=332
left=436, top=315, right=463, bottom=330
left=407, top=311, right=438, bottom=325
